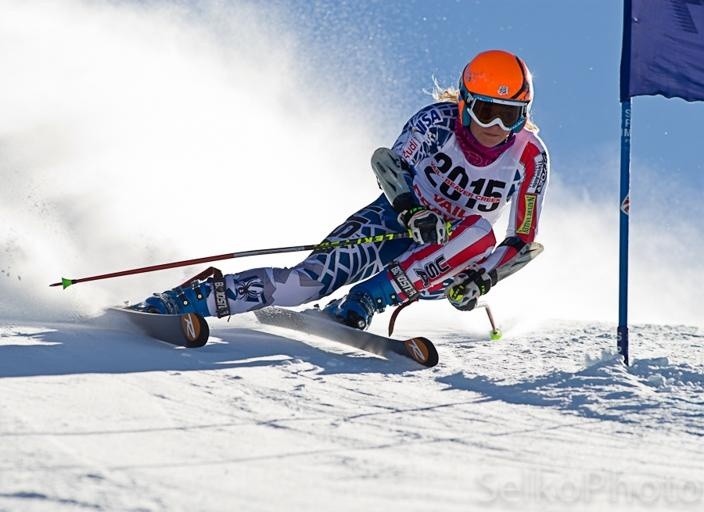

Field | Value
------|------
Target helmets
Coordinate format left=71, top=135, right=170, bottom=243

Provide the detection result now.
left=458, top=50, right=534, bottom=126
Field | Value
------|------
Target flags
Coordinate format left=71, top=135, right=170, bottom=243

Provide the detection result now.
left=620, top=0, right=703, bottom=102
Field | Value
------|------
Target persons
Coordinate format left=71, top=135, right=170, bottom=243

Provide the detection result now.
left=123, top=50, right=550, bottom=331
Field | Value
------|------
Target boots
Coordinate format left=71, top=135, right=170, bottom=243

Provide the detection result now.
left=146, top=282, right=211, bottom=319
left=319, top=271, right=402, bottom=330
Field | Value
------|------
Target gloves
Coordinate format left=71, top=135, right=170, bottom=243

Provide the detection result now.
left=402, top=206, right=446, bottom=245
left=445, top=263, right=490, bottom=310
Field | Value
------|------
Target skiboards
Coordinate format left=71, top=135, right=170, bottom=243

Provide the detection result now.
left=102, top=306, right=439, bottom=367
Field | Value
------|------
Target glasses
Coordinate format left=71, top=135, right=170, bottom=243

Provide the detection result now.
left=465, top=96, right=528, bottom=131
